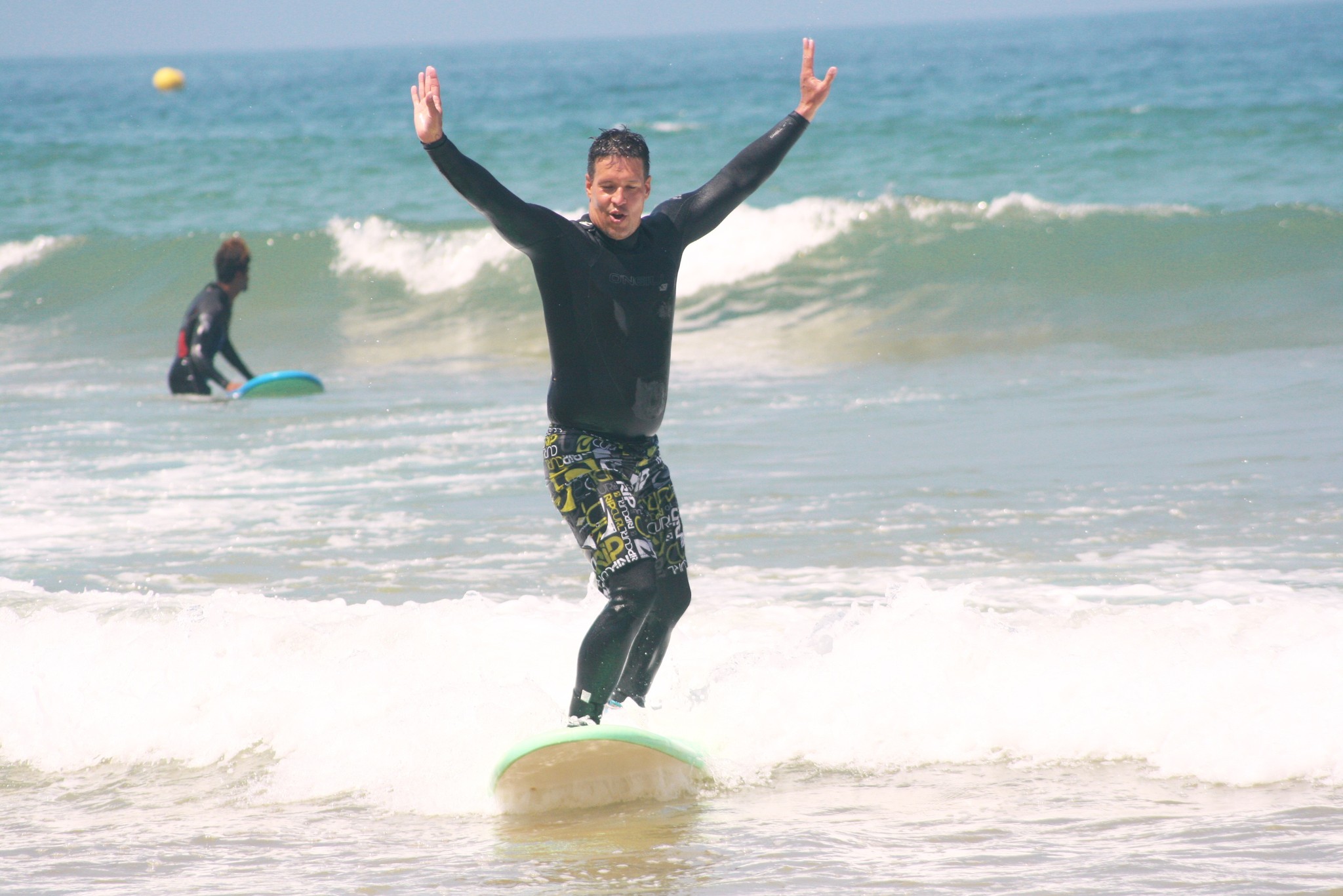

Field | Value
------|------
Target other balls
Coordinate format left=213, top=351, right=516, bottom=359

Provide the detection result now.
left=152, top=66, right=186, bottom=92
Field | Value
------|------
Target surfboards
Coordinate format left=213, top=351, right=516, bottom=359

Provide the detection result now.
left=490, top=729, right=715, bottom=814
left=211, top=370, right=326, bottom=401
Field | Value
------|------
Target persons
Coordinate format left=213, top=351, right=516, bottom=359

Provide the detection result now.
left=409, top=36, right=838, bottom=732
left=168, top=237, right=255, bottom=397
